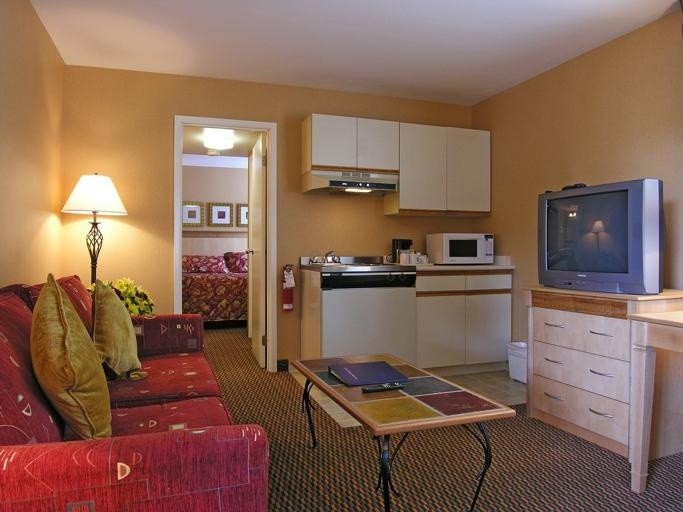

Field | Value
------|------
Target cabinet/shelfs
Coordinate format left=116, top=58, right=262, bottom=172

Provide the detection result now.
left=301, top=113, right=399, bottom=195
left=383, top=122, right=491, bottom=217
left=415, top=266, right=517, bottom=369
left=523, top=287, right=683, bottom=462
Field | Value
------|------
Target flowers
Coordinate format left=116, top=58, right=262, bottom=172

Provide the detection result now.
left=88, top=278, right=155, bottom=313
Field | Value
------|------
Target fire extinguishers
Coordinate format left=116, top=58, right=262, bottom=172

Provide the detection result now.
left=282, top=264, right=295, bottom=311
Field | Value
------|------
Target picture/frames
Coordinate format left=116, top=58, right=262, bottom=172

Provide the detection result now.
left=237, top=203, right=248, bottom=227
left=208, top=202, right=233, bottom=227
left=182, top=201, right=204, bottom=227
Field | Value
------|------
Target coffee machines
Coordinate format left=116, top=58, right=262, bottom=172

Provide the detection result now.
left=392, top=239, right=413, bottom=262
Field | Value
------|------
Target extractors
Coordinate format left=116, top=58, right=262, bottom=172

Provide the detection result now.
left=302, top=171, right=398, bottom=195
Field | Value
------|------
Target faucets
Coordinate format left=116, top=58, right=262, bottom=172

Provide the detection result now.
left=324, top=249, right=336, bottom=263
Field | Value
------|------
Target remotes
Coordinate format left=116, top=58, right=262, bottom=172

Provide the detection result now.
left=360, top=380, right=406, bottom=395
left=561, top=182, right=586, bottom=191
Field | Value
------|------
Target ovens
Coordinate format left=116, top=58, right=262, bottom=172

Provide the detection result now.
left=319, top=268, right=417, bottom=367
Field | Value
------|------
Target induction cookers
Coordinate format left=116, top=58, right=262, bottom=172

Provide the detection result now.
left=343, top=256, right=399, bottom=266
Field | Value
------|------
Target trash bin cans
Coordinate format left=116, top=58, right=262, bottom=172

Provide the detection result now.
left=506, top=341, right=528, bottom=384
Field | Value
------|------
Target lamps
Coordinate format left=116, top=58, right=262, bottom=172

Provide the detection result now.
left=61, top=170, right=129, bottom=287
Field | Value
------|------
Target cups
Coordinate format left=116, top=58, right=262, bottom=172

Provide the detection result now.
left=399, top=254, right=409, bottom=265
left=410, top=255, right=420, bottom=264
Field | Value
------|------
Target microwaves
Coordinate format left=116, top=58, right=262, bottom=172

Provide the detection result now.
left=426, top=233, right=493, bottom=265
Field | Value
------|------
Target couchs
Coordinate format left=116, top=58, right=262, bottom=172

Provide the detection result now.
left=0, top=274, right=270, bottom=511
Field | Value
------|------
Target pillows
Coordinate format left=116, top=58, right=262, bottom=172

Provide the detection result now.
left=30, top=273, right=113, bottom=440
left=21, top=274, right=93, bottom=339
left=182, top=254, right=228, bottom=272
left=182, top=255, right=229, bottom=273
left=224, top=251, right=248, bottom=273
left=93, top=279, right=142, bottom=380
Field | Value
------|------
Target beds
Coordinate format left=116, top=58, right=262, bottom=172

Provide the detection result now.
left=182, top=272, right=248, bottom=322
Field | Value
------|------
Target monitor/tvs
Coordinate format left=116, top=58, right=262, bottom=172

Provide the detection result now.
left=536, top=176, right=665, bottom=296
